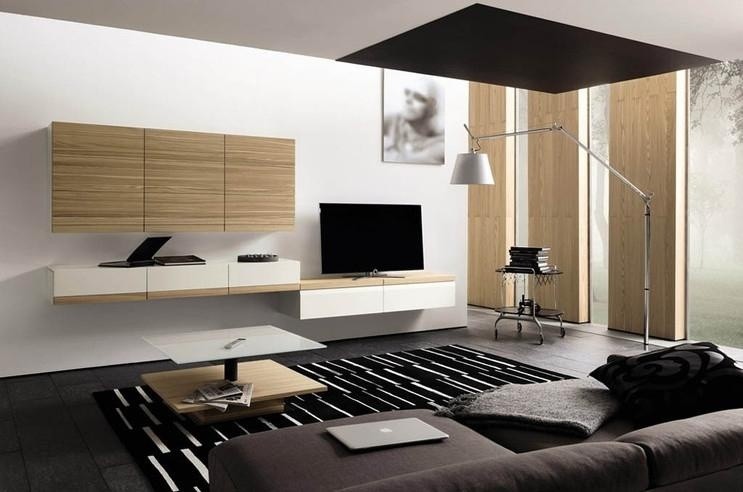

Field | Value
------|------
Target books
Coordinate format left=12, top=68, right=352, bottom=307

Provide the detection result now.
left=98, top=235, right=206, bottom=269
left=504, top=246, right=552, bottom=274
left=181, top=379, right=255, bottom=413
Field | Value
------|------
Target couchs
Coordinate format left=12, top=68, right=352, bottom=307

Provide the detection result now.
left=207, top=355, right=743, bottom=491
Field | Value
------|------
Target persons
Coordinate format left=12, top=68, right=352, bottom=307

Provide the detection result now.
left=383, top=76, right=445, bottom=163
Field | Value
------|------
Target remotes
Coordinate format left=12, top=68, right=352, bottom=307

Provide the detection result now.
left=224, top=337, right=247, bottom=349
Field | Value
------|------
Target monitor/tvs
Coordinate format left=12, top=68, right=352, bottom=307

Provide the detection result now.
left=318, top=202, right=425, bottom=278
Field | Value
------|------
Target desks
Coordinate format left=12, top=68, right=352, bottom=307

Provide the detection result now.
left=492, top=264, right=567, bottom=345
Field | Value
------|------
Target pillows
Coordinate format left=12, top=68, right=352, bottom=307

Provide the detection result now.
left=587, top=340, right=742, bottom=428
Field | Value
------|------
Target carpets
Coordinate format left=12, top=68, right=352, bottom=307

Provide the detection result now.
left=91, top=343, right=576, bottom=492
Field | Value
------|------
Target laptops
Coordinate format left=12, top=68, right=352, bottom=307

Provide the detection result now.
left=325, top=417, right=450, bottom=451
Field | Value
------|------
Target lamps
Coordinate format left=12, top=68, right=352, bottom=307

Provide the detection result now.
left=449, top=122, right=655, bottom=345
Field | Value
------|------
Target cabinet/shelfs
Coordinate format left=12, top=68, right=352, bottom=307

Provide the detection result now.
left=45, top=121, right=298, bottom=234
